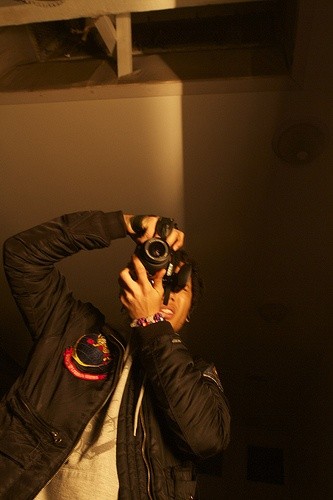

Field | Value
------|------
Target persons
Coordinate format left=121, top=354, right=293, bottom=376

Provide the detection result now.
left=0, top=209, right=233, bottom=500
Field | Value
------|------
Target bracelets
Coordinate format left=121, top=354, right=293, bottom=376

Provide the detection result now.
left=129, top=312, right=165, bottom=328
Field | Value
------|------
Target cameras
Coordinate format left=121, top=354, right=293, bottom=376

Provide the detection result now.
left=128, top=237, right=192, bottom=293
